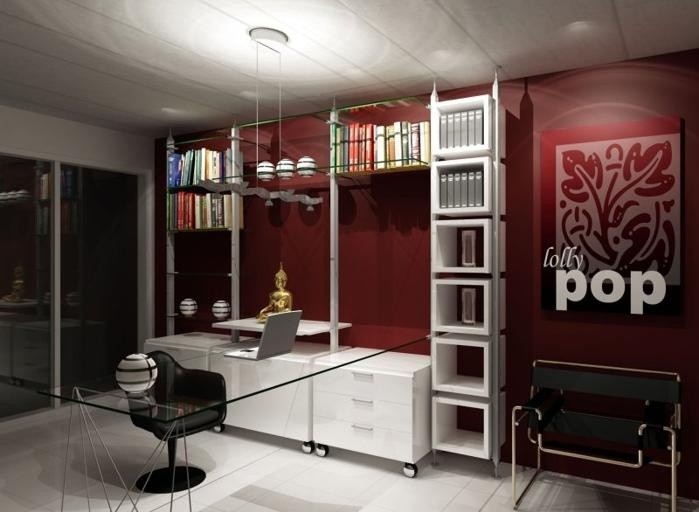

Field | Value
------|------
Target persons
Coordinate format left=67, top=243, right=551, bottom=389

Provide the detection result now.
left=258, top=261, right=293, bottom=323
left=4, top=260, right=25, bottom=302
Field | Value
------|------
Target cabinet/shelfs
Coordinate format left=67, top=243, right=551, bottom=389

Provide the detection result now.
left=333, top=160, right=430, bottom=185
left=430, top=68, right=507, bottom=479
left=165, top=151, right=232, bottom=231
left=0, top=318, right=101, bottom=389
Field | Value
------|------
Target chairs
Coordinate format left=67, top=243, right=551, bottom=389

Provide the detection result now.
left=511, top=359, right=683, bottom=512
left=127, top=349, right=225, bottom=495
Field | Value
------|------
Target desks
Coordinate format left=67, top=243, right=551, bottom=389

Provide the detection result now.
left=34, top=329, right=427, bottom=512
left=212, top=315, right=352, bottom=338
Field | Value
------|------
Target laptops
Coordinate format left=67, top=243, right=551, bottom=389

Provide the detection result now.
left=224, top=310, right=303, bottom=361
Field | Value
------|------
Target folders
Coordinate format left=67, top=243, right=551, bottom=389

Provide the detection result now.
left=440, top=170, right=482, bottom=208
left=440, top=109, right=482, bottom=148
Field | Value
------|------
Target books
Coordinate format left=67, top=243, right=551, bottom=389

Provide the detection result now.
left=168, top=150, right=244, bottom=233
left=37, top=166, right=79, bottom=235
left=436, top=107, right=484, bottom=210
left=336, top=120, right=429, bottom=171
